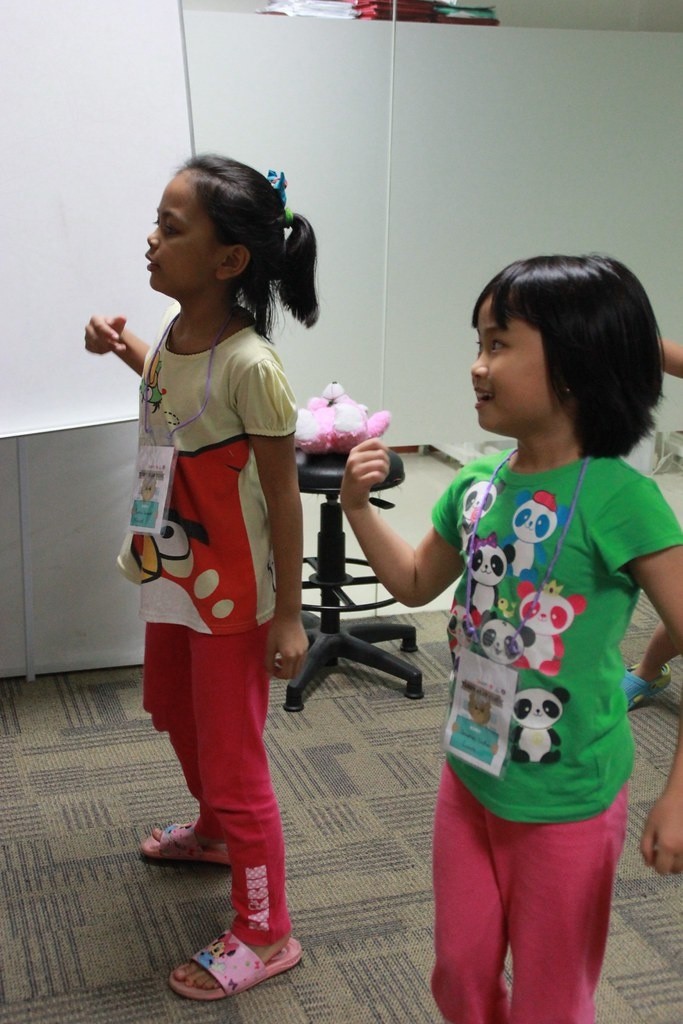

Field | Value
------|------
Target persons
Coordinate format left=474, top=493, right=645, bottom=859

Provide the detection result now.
left=340, top=255, right=683, bottom=1024
left=85, top=154, right=318, bottom=1001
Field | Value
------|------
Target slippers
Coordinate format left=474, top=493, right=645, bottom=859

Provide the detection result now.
left=621, top=663, right=671, bottom=709
left=169, top=929, right=302, bottom=1001
left=140, top=820, right=230, bottom=865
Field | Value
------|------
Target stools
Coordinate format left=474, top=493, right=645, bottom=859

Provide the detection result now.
left=282, top=447, right=424, bottom=711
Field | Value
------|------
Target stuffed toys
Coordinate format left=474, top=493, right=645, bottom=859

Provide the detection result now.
left=294, top=381, right=391, bottom=454
